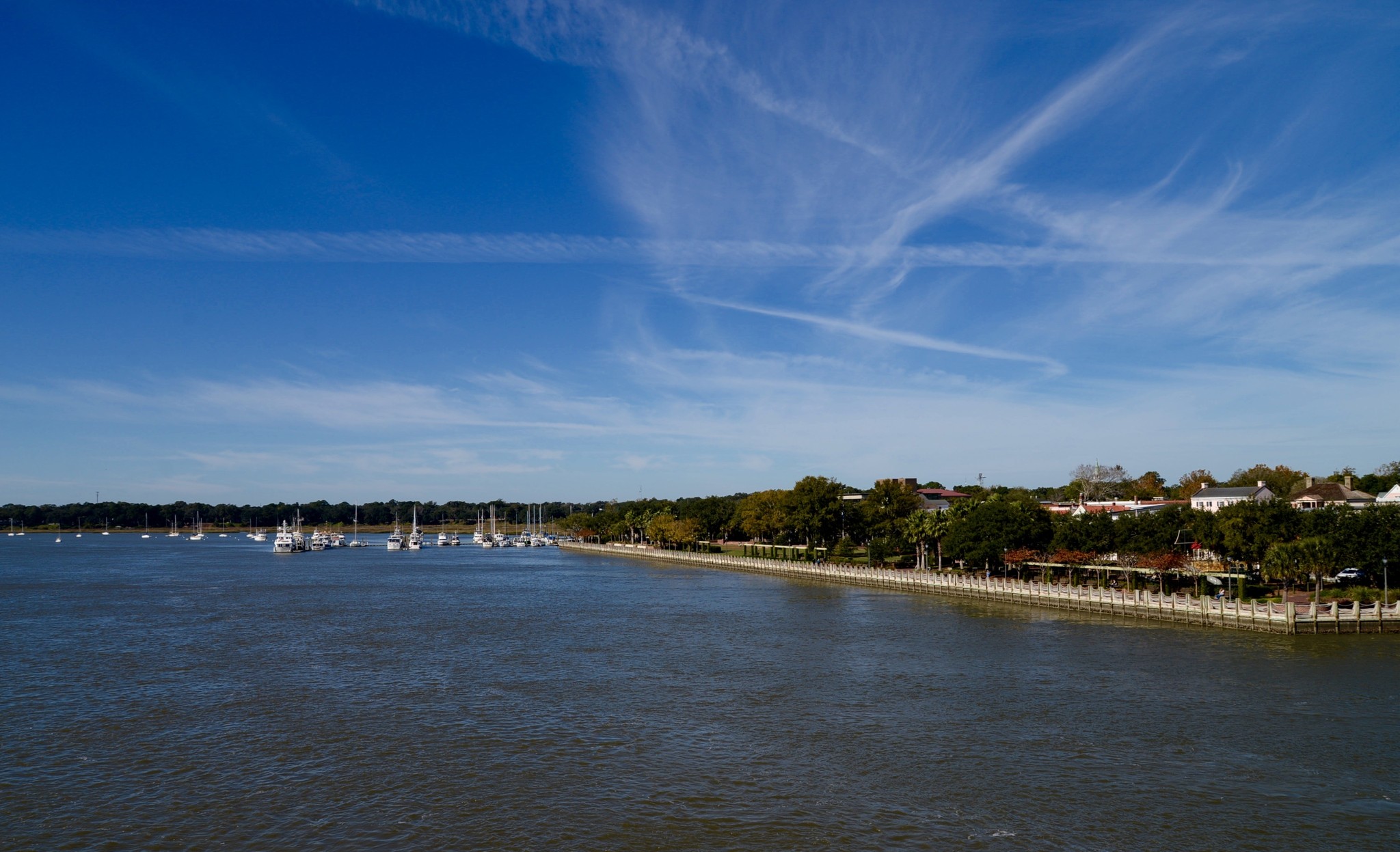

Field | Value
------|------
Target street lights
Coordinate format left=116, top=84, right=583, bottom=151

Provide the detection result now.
left=1382, top=556, right=1390, bottom=608
left=1004, top=546, right=1008, bottom=583
left=867, top=541, right=871, bottom=570
left=788, top=539, right=791, bottom=563
left=610, top=531, right=612, bottom=550
left=925, top=543, right=929, bottom=575
left=645, top=536, right=647, bottom=551
left=1226, top=554, right=1233, bottom=603
left=815, top=540, right=818, bottom=565
left=725, top=536, right=728, bottom=560
left=668, top=534, right=671, bottom=552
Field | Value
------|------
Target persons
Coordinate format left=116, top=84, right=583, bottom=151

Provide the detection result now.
left=1215, top=587, right=1225, bottom=599
left=817, top=558, right=820, bottom=566
left=1107, top=579, right=1118, bottom=588
left=986, top=570, right=990, bottom=577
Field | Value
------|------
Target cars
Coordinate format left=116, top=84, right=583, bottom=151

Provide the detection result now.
left=1335, top=566, right=1365, bottom=584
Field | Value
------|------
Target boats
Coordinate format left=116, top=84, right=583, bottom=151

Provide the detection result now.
left=246, top=517, right=268, bottom=542
left=272, top=499, right=362, bottom=554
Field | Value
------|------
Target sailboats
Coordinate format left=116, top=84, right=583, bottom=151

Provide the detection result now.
left=386, top=503, right=424, bottom=551
left=437, top=513, right=447, bottom=546
left=141, top=512, right=151, bottom=538
left=75, top=517, right=82, bottom=537
left=218, top=518, right=228, bottom=537
left=165, top=510, right=205, bottom=541
left=16, top=520, right=25, bottom=536
left=55, top=525, right=62, bottom=542
left=102, top=516, right=111, bottom=535
left=472, top=503, right=584, bottom=547
left=8, top=519, right=15, bottom=536
left=448, top=530, right=460, bottom=546
